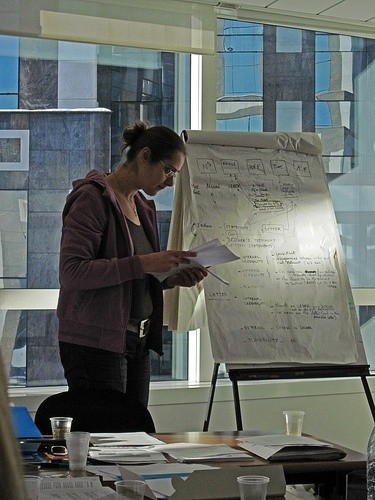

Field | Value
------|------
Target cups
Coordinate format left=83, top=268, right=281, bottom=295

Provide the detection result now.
left=114, top=479, right=146, bottom=500
left=64, top=431, right=91, bottom=470
left=237, top=475, right=269, bottom=500
left=283, top=410, right=305, bottom=437
left=50, top=417, right=73, bottom=439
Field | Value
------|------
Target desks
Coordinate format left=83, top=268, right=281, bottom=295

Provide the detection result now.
left=43, top=427, right=367, bottom=500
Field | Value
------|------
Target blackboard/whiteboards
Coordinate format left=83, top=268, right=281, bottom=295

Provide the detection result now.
left=180, top=127, right=372, bottom=382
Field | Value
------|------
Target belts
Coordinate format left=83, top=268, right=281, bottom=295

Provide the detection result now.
left=128, top=318, right=152, bottom=338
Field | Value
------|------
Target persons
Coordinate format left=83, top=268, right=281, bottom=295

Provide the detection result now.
left=34, top=117, right=207, bottom=436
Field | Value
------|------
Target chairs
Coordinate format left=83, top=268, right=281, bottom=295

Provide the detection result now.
left=34, top=390, right=155, bottom=434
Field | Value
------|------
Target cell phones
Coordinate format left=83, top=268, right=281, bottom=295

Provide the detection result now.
left=50, top=446, right=67, bottom=455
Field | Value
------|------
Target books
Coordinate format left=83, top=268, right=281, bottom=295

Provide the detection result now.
left=9, top=405, right=44, bottom=465
left=269, top=445, right=347, bottom=462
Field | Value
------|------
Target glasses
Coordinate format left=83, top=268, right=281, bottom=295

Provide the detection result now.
left=159, top=159, right=177, bottom=178
